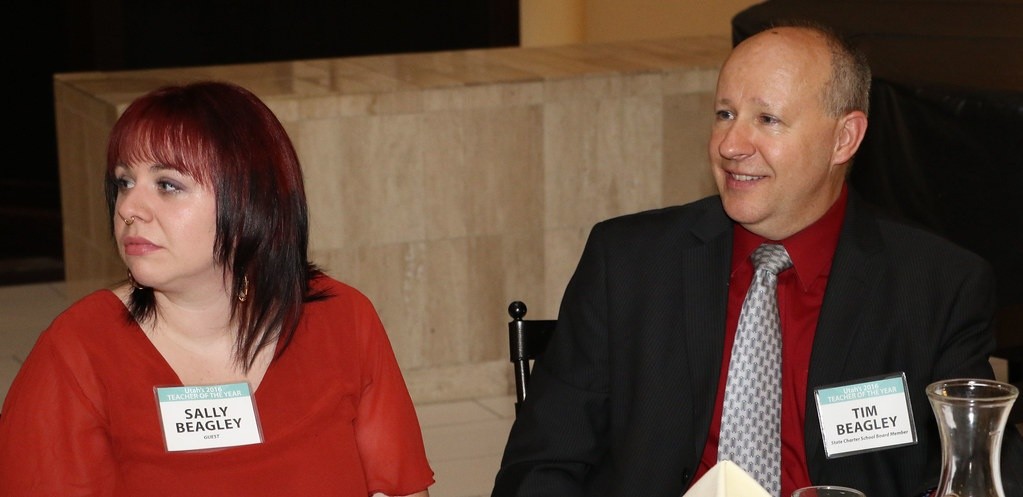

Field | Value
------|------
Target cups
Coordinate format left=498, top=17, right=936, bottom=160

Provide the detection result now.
left=790, top=485, right=867, bottom=497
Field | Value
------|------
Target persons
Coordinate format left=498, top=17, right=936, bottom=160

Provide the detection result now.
left=492, top=21, right=1023, bottom=497
left=0, top=80, right=436, bottom=497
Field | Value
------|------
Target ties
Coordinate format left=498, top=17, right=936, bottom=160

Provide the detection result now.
left=716, top=243, right=794, bottom=497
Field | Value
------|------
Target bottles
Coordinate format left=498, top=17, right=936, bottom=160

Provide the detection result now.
left=925, top=378, right=1019, bottom=497
